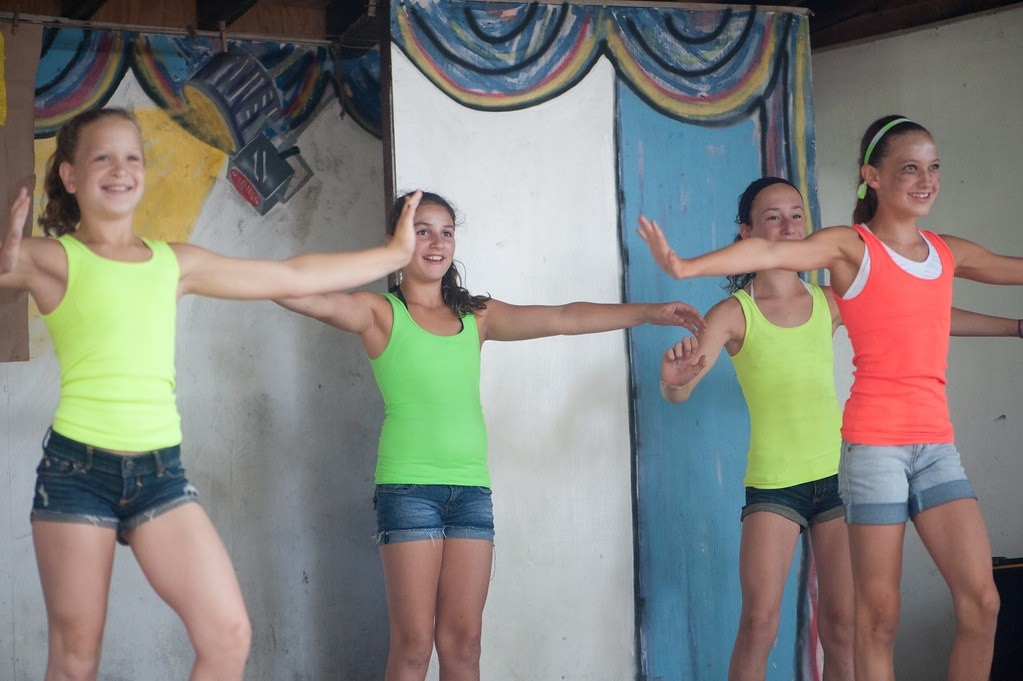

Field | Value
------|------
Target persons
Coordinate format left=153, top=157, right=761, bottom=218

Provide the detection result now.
left=633, top=116, right=1022, bottom=681
left=659, top=175, right=1023, bottom=679
left=2, top=106, right=423, bottom=681
left=275, top=190, right=705, bottom=680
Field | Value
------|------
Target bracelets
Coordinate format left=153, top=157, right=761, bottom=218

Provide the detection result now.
left=1017, top=319, right=1023, bottom=339
